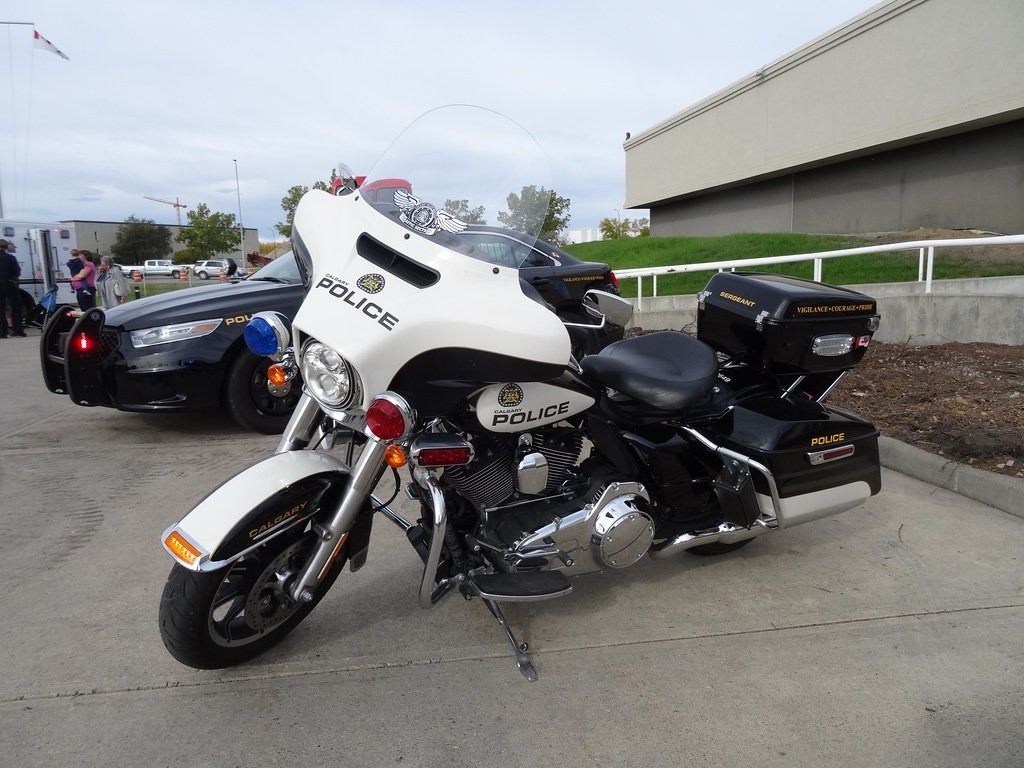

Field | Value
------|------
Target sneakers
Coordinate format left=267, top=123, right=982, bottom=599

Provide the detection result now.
left=70, top=290, right=75, bottom=293
left=83, top=288, right=93, bottom=296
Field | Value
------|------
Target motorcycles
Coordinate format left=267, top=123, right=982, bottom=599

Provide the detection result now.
left=156, top=103, right=887, bottom=669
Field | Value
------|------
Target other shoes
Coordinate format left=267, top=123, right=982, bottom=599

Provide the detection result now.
left=7, top=328, right=27, bottom=338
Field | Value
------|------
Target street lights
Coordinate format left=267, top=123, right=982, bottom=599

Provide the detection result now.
left=265, top=227, right=277, bottom=262
left=232, top=157, right=249, bottom=269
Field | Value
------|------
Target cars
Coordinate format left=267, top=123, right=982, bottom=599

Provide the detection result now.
left=118, top=257, right=251, bottom=281
left=40, top=226, right=627, bottom=433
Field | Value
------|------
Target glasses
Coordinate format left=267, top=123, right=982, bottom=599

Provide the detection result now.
left=101, top=263, right=107, bottom=267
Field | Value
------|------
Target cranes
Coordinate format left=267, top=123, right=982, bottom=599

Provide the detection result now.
left=145, top=192, right=187, bottom=225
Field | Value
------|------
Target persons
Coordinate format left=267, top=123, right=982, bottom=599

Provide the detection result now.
left=0, top=239, right=36, bottom=340
left=68, top=250, right=97, bottom=311
left=97, top=256, right=127, bottom=308
left=67, top=249, right=92, bottom=295
